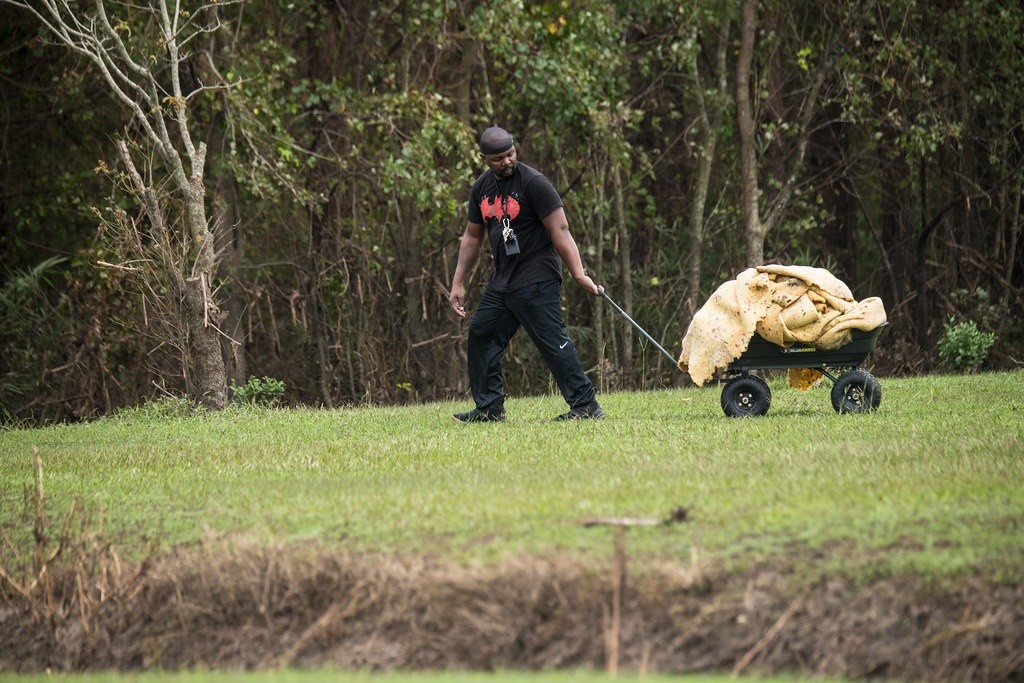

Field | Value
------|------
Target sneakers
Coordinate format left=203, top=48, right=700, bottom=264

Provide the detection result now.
left=452, top=408, right=507, bottom=424
left=554, top=404, right=607, bottom=421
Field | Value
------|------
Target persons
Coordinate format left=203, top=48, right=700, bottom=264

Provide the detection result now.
left=449, top=127, right=608, bottom=424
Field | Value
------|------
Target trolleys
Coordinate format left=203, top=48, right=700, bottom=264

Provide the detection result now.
left=597, top=290, right=888, bottom=416
